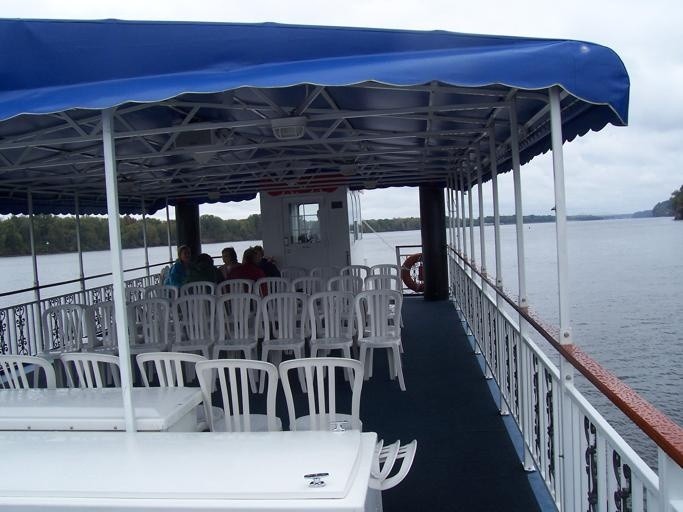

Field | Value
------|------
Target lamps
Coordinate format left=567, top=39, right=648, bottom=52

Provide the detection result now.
left=270, top=105, right=309, bottom=141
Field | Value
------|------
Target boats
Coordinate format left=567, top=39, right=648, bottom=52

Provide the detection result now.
left=1, top=19, right=682, bottom=512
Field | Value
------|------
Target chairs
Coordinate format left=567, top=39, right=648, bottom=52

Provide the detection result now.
left=134, top=351, right=225, bottom=432
left=59, top=351, right=123, bottom=389
left=0, top=354, right=57, bottom=390
left=277, top=356, right=366, bottom=433
left=194, top=358, right=284, bottom=433
left=366, top=438, right=418, bottom=490
left=26, top=263, right=407, bottom=392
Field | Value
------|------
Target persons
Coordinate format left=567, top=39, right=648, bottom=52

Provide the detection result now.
left=162, top=244, right=281, bottom=312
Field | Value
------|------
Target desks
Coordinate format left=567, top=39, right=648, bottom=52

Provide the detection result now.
left=1, top=386, right=204, bottom=433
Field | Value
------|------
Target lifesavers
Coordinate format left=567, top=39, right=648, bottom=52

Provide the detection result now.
left=401, top=253, right=424, bottom=292
